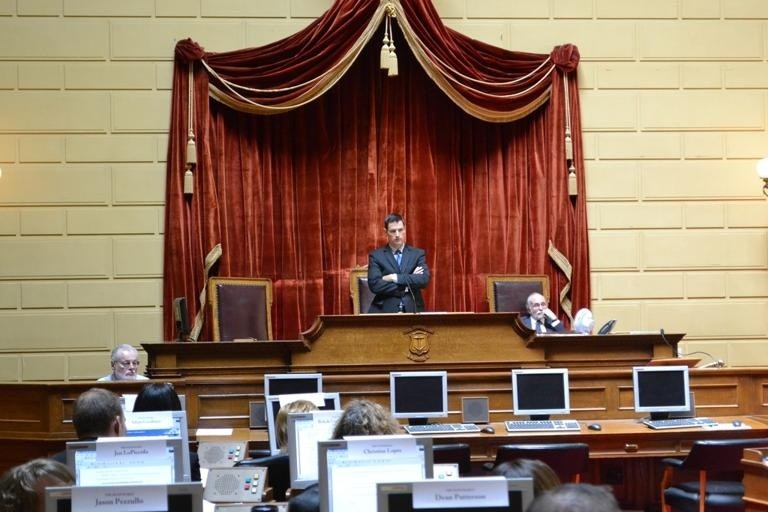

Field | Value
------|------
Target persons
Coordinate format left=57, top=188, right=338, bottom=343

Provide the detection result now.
left=134, top=381, right=182, bottom=416
left=527, top=485, right=622, bottom=510
left=367, top=214, right=430, bottom=313
left=495, top=462, right=558, bottom=499
left=520, top=292, right=565, bottom=334
left=291, top=398, right=403, bottom=511
left=48, top=389, right=126, bottom=459
left=98, top=345, right=151, bottom=382
left=275, top=399, right=318, bottom=454
left=1, top=456, right=74, bottom=511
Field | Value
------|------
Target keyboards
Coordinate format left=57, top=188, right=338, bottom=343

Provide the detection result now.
left=504, top=420, right=580, bottom=431
left=644, top=417, right=718, bottom=430
left=403, top=423, right=481, bottom=435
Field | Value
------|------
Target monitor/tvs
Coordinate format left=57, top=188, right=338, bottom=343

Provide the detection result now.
left=318, top=436, right=434, bottom=511
left=512, top=369, right=570, bottom=420
left=264, top=373, right=322, bottom=421
left=122, top=393, right=185, bottom=412
left=433, top=442, right=470, bottom=480
left=265, top=391, right=341, bottom=456
left=46, top=482, right=203, bottom=510
left=66, top=436, right=183, bottom=487
left=124, top=411, right=191, bottom=483
left=375, top=477, right=531, bottom=511
left=287, top=410, right=345, bottom=489
left=633, top=366, right=690, bottom=419
left=389, top=372, right=448, bottom=419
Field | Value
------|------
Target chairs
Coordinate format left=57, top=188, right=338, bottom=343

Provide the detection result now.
left=486, top=275, right=550, bottom=320
left=350, top=270, right=376, bottom=314
left=208, top=277, right=274, bottom=342
left=659, top=437, right=768, bottom=512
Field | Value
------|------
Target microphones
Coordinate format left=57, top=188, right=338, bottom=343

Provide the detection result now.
left=402, top=274, right=417, bottom=313
left=660, top=329, right=724, bottom=368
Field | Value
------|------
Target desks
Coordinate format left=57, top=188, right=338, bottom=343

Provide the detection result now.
left=196, top=416, right=767, bottom=461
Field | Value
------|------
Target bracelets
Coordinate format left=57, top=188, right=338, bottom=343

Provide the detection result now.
left=552, top=318, right=558, bottom=322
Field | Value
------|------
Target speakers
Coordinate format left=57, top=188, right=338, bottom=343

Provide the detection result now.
left=670, top=391, right=696, bottom=418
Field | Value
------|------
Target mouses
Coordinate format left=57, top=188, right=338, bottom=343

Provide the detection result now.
left=733, top=421, right=741, bottom=427
left=589, top=424, right=601, bottom=430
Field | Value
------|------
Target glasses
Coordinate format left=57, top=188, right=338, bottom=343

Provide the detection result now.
left=114, top=359, right=140, bottom=365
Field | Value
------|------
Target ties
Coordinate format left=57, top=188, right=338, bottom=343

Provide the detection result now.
left=535, top=320, right=543, bottom=334
left=396, top=251, right=402, bottom=265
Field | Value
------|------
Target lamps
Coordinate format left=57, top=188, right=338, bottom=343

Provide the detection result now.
left=756, top=158, right=768, bottom=196
